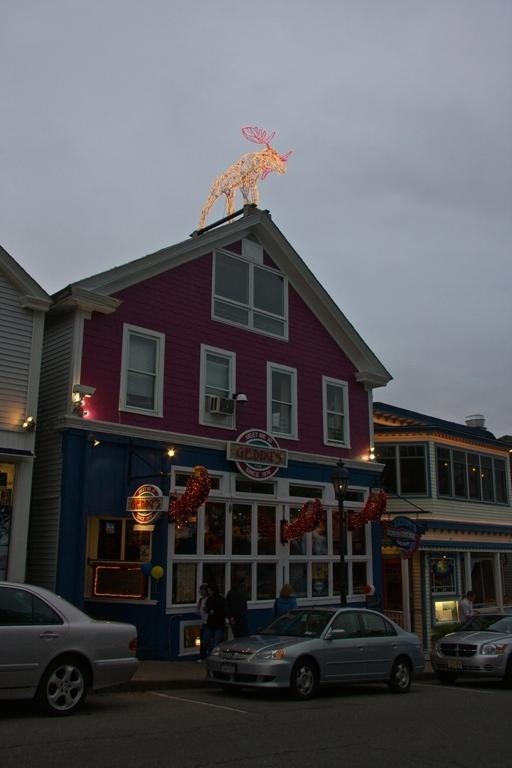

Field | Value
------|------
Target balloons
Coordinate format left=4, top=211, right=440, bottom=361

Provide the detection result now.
left=150, top=565, right=164, bottom=581
left=369, top=584, right=375, bottom=595
left=362, top=584, right=371, bottom=593
left=140, top=563, right=153, bottom=577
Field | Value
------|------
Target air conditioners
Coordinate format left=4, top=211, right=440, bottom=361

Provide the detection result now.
left=209, top=396, right=236, bottom=416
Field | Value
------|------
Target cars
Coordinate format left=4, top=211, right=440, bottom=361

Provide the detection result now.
left=431, top=610, right=511, bottom=689
left=1, top=580, right=141, bottom=718
left=205, top=606, right=425, bottom=702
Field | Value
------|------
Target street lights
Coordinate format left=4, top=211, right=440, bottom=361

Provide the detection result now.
left=329, top=457, right=352, bottom=607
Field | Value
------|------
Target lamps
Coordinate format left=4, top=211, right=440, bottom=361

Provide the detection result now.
left=91, top=438, right=102, bottom=448
left=23, top=416, right=37, bottom=432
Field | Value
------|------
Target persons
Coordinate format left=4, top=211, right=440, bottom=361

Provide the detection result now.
left=272, top=583, right=297, bottom=616
left=225, top=589, right=248, bottom=639
left=202, top=582, right=226, bottom=660
left=195, top=584, right=211, bottom=664
left=460, top=591, right=476, bottom=625
